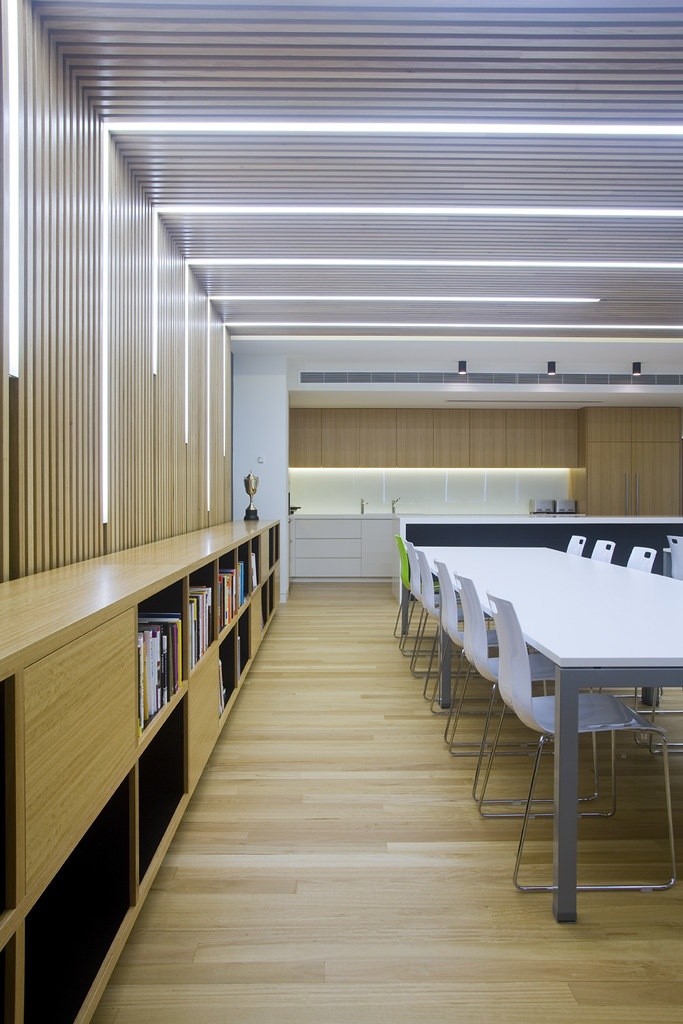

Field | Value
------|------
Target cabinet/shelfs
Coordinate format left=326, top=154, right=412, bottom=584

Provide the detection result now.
left=1, top=518, right=283, bottom=1024
left=289, top=518, right=400, bottom=581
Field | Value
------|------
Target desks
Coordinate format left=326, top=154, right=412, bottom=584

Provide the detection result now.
left=414, top=544, right=683, bottom=925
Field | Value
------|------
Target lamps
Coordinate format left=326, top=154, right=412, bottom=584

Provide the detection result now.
left=458, top=360, right=467, bottom=375
left=548, top=361, right=556, bottom=376
left=632, top=362, right=642, bottom=376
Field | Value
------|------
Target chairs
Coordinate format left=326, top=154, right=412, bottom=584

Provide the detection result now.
left=565, top=536, right=588, bottom=556
left=666, top=535, right=683, bottom=582
left=398, top=541, right=443, bottom=658
left=409, top=548, right=465, bottom=680
left=442, top=573, right=554, bottom=757
left=589, top=539, right=617, bottom=564
left=424, top=560, right=501, bottom=704
left=393, top=535, right=438, bottom=653
left=472, top=591, right=677, bottom=893
left=626, top=547, right=658, bottom=573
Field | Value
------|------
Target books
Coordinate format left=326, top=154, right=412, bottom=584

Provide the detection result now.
left=138, top=551, right=261, bottom=732
